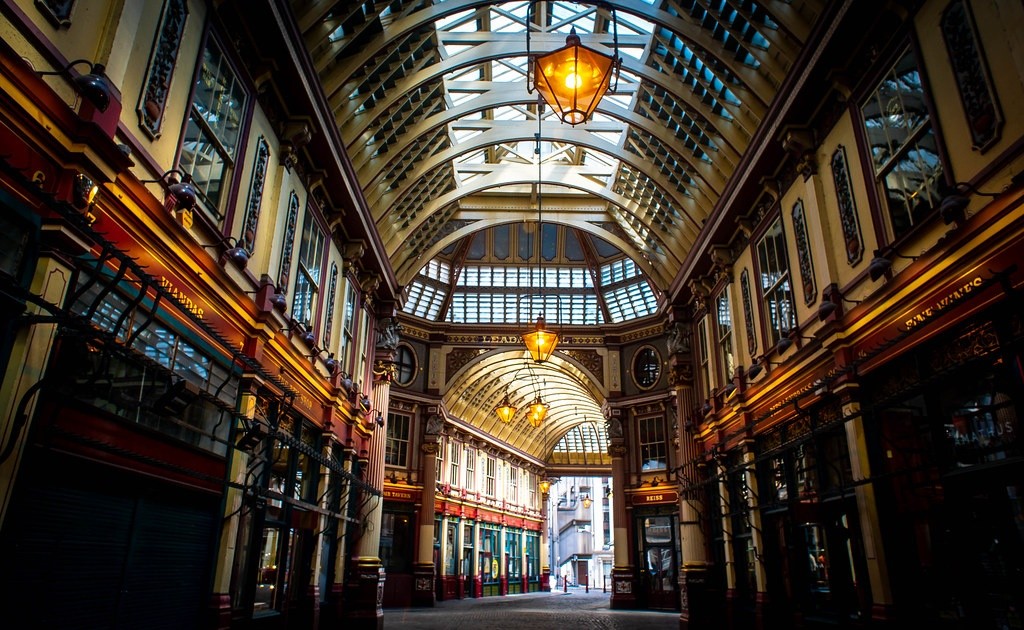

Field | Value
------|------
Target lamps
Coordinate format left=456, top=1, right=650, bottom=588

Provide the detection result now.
left=34, top=59, right=110, bottom=113
left=495, top=223, right=550, bottom=428
left=235, top=416, right=269, bottom=453
left=683, top=282, right=863, bottom=434
left=153, top=375, right=201, bottom=423
left=280, top=322, right=384, bottom=427
left=939, top=183, right=1002, bottom=226
left=140, top=169, right=197, bottom=213
left=869, top=247, right=922, bottom=282
left=580, top=416, right=592, bottom=509
left=538, top=417, right=551, bottom=495
left=244, top=283, right=287, bottom=314
left=201, top=236, right=248, bottom=272
left=526, top=0, right=623, bottom=128
left=518, top=92, right=562, bottom=364
